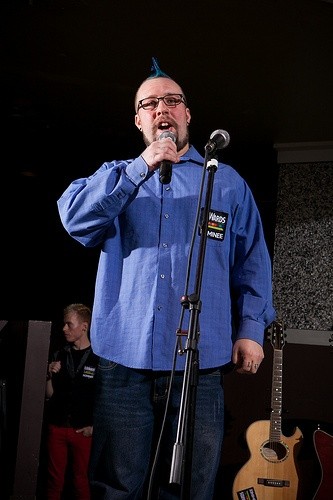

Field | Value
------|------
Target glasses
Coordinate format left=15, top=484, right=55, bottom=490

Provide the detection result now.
left=137, top=94, right=187, bottom=112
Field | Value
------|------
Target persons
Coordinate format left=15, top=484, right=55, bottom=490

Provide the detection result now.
left=46, top=304, right=101, bottom=500
left=56, top=57, right=276, bottom=500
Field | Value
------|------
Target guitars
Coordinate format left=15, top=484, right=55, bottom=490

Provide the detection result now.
left=234, top=318, right=302, bottom=494
left=312, top=428, right=333, bottom=494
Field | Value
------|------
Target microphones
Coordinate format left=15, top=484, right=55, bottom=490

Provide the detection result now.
left=158, top=131, right=176, bottom=184
left=205, top=129, right=230, bottom=152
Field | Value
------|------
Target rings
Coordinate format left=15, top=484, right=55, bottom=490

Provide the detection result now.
left=253, top=364, right=258, bottom=369
left=247, top=362, right=251, bottom=368
